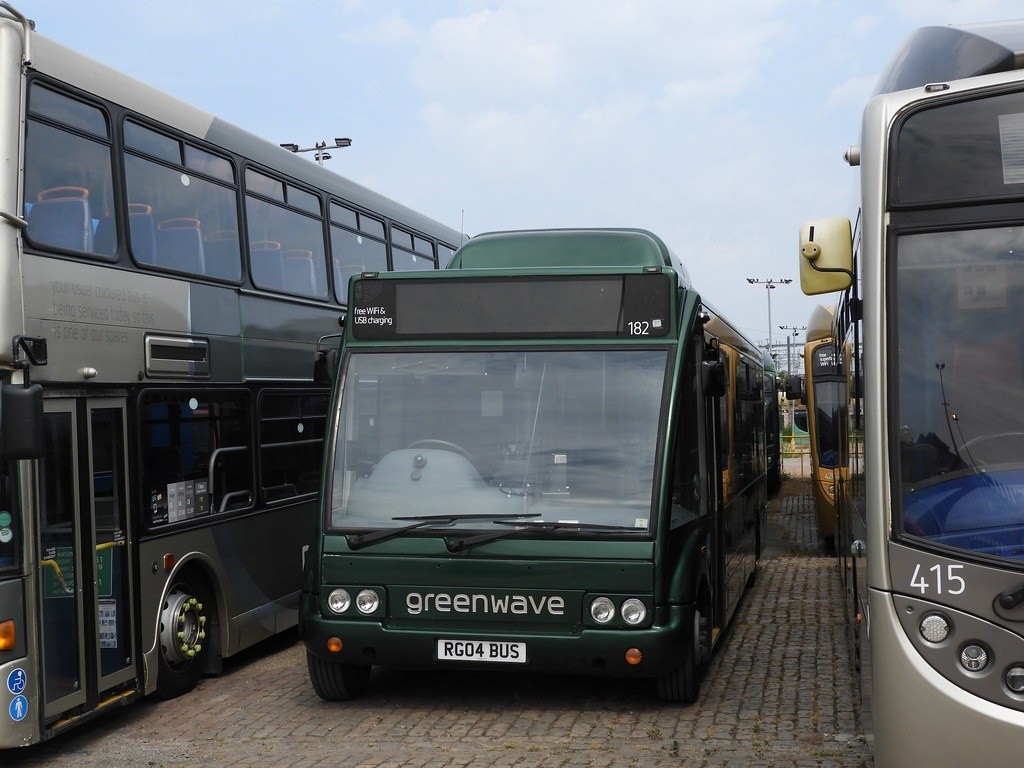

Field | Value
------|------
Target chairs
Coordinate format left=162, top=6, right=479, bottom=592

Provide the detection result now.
left=31, top=186, right=317, bottom=302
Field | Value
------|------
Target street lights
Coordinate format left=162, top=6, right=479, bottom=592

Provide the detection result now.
left=951, top=414, right=976, bottom=468
left=934, top=362, right=965, bottom=464
left=280, top=137, right=352, bottom=167
left=778, top=326, right=807, bottom=375
left=747, top=277, right=794, bottom=356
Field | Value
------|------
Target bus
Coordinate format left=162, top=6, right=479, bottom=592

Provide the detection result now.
left=785, top=303, right=850, bottom=543
left=798, top=27, right=1024, bottom=768
left=299, top=226, right=780, bottom=707
left=1, top=2, right=475, bottom=753
